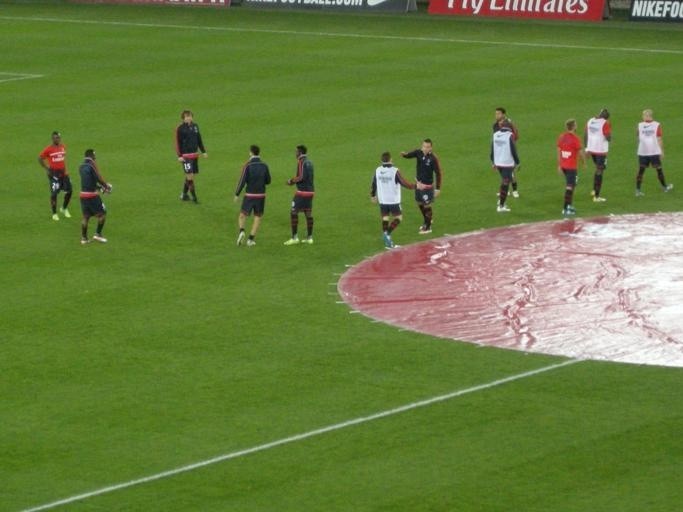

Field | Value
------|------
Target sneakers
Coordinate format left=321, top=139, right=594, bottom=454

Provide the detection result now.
left=496, top=190, right=520, bottom=212
left=636, top=192, right=645, bottom=197
left=237, top=231, right=314, bottom=246
left=562, top=206, right=577, bottom=217
left=664, top=184, right=673, bottom=192
left=384, top=231, right=394, bottom=249
left=53, top=206, right=108, bottom=245
left=591, top=191, right=606, bottom=202
left=181, top=193, right=200, bottom=204
left=419, top=220, right=433, bottom=235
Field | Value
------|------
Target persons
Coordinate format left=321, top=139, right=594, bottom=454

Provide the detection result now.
left=78, top=148, right=111, bottom=245
left=635, top=108, right=673, bottom=198
left=399, top=138, right=442, bottom=235
left=584, top=107, right=612, bottom=203
left=232, top=145, right=272, bottom=246
left=491, top=107, right=586, bottom=216
left=369, top=151, right=427, bottom=248
left=37, top=131, right=73, bottom=221
left=174, top=110, right=208, bottom=204
left=283, top=144, right=315, bottom=245
